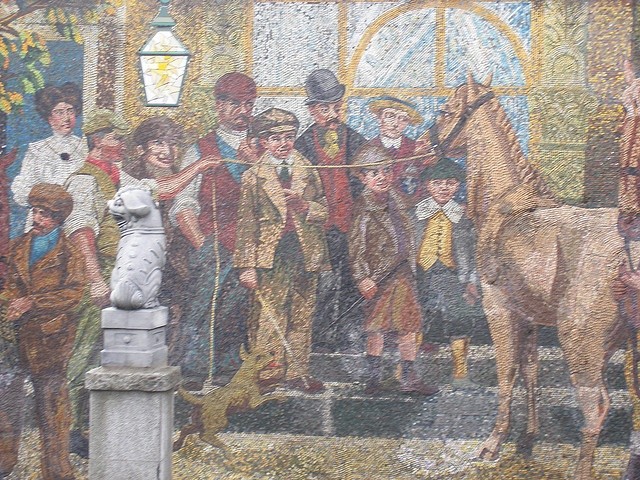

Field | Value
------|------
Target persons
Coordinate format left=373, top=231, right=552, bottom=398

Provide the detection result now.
left=0, top=183, right=87, bottom=479
left=169, top=73, right=258, bottom=386
left=347, top=148, right=440, bottom=397
left=291, top=69, right=369, bottom=354
left=62, top=108, right=221, bottom=462
left=407, top=158, right=484, bottom=393
left=231, top=108, right=331, bottom=394
left=610, top=31, right=640, bottom=471
left=11, top=83, right=89, bottom=233
left=132, top=117, right=192, bottom=363
left=365, top=93, right=431, bottom=206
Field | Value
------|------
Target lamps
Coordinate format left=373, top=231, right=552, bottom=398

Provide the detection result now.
left=136, top=0, right=191, bottom=108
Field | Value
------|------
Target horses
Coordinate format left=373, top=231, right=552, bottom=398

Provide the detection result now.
left=615, top=59, right=640, bottom=245
left=413, top=68, right=635, bottom=480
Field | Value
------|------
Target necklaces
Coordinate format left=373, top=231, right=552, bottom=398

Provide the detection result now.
left=42, top=137, right=84, bottom=161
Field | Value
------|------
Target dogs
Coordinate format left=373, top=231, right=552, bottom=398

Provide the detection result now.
left=170, top=342, right=291, bottom=462
